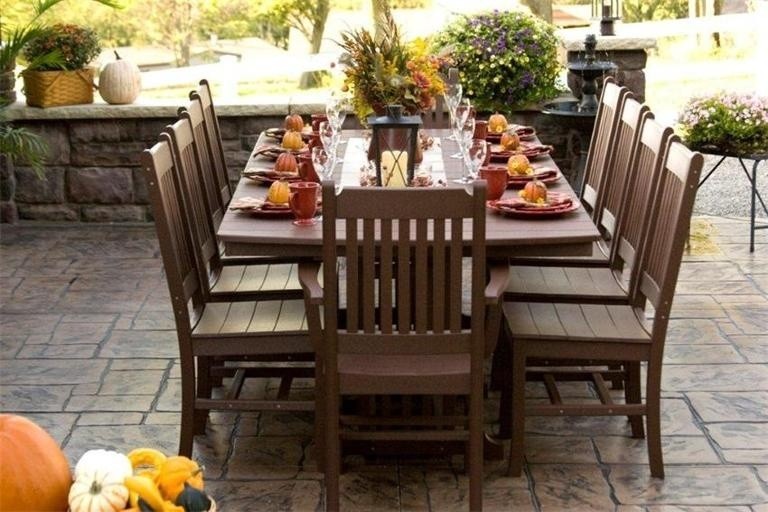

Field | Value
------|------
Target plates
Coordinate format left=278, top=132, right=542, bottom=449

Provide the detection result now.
left=247, top=170, right=309, bottom=188
left=265, top=128, right=327, bottom=142
left=481, top=162, right=562, bottom=185
left=235, top=201, right=321, bottom=217
left=472, top=126, right=536, bottom=143
left=256, top=147, right=325, bottom=167
left=486, top=193, right=580, bottom=217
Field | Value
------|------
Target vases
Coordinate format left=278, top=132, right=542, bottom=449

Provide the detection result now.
left=366, top=128, right=425, bottom=164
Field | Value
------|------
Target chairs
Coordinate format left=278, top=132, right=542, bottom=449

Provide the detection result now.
left=510, top=91, right=652, bottom=388
left=177, top=99, right=317, bottom=261
left=297, top=182, right=510, bottom=512
left=578, top=75, right=629, bottom=223
left=499, top=136, right=704, bottom=480
left=503, top=110, right=674, bottom=440
left=164, top=112, right=339, bottom=457
left=187, top=78, right=235, bottom=210
left=140, top=130, right=327, bottom=473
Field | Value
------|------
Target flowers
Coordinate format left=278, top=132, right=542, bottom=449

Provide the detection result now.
left=671, top=86, right=768, bottom=152
left=429, top=6, right=569, bottom=111
left=19, top=15, right=108, bottom=72
left=324, top=0, right=454, bottom=117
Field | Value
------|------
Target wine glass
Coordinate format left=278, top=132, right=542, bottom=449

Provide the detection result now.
left=442, top=83, right=464, bottom=141
left=311, top=148, right=337, bottom=222
left=326, top=102, right=347, bottom=159
left=319, top=119, right=339, bottom=181
left=458, top=140, right=488, bottom=186
left=452, top=117, right=475, bottom=187
left=450, top=99, right=471, bottom=160
left=486, top=140, right=554, bottom=161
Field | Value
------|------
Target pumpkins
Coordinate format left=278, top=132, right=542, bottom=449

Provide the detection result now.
left=175, top=481, right=211, bottom=511
left=274, top=148, right=297, bottom=171
left=267, top=175, right=290, bottom=204
left=0, top=410, right=72, bottom=512
left=500, top=128, right=520, bottom=150
left=508, top=147, right=529, bottom=176
left=489, top=110, right=508, bottom=132
left=281, top=126, right=302, bottom=150
left=523, top=173, right=547, bottom=203
left=75, top=449, right=133, bottom=480
left=285, top=112, right=303, bottom=132
left=68, top=467, right=129, bottom=512
left=160, top=455, right=206, bottom=502
left=98, top=50, right=142, bottom=105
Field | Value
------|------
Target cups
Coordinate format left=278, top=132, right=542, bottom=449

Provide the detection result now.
left=293, top=156, right=319, bottom=184
left=311, top=114, right=330, bottom=140
left=455, top=106, right=476, bottom=121
left=289, top=181, right=316, bottom=228
left=469, top=140, right=491, bottom=167
left=473, top=167, right=509, bottom=202
left=466, top=120, right=488, bottom=145
left=308, top=130, right=326, bottom=154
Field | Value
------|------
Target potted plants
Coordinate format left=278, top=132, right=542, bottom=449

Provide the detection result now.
left=0, top=0, right=130, bottom=106
